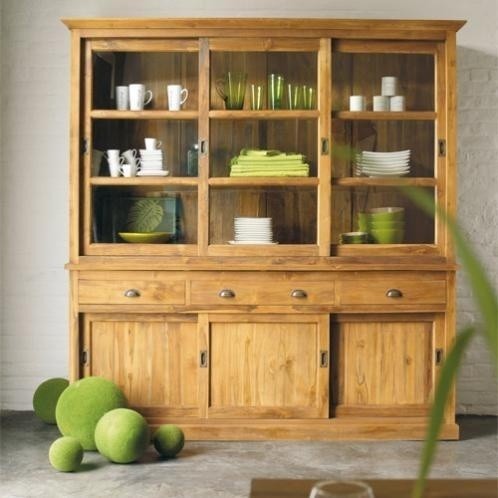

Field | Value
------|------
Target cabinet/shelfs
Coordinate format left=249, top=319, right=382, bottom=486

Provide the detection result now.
left=62, top=17, right=467, bottom=441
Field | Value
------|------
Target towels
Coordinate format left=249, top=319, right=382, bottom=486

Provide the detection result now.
left=229, top=149, right=310, bottom=178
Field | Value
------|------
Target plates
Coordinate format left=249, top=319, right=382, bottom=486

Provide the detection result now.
left=227, top=218, right=279, bottom=245
left=353, top=150, right=413, bottom=176
left=139, top=150, right=168, bottom=177
left=118, top=232, right=173, bottom=244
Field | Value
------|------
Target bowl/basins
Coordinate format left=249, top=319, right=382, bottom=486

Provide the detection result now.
left=341, top=232, right=366, bottom=244
left=356, top=206, right=407, bottom=244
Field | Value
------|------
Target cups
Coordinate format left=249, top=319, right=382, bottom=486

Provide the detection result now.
left=123, top=149, right=137, bottom=162
left=287, top=85, right=304, bottom=110
left=116, top=85, right=129, bottom=109
left=305, top=89, right=315, bottom=110
left=380, top=77, right=399, bottom=97
left=267, top=74, right=285, bottom=110
left=215, top=73, right=246, bottom=109
left=128, top=83, right=150, bottom=111
left=307, top=477, right=372, bottom=498
left=166, top=85, right=188, bottom=111
left=349, top=95, right=367, bottom=111
left=373, top=96, right=389, bottom=111
left=106, top=151, right=121, bottom=180
left=389, top=96, right=404, bottom=112
left=250, top=85, right=262, bottom=110
left=121, top=164, right=138, bottom=176
left=144, top=138, right=162, bottom=149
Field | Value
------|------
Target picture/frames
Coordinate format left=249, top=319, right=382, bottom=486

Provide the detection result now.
left=109, top=195, right=180, bottom=241
